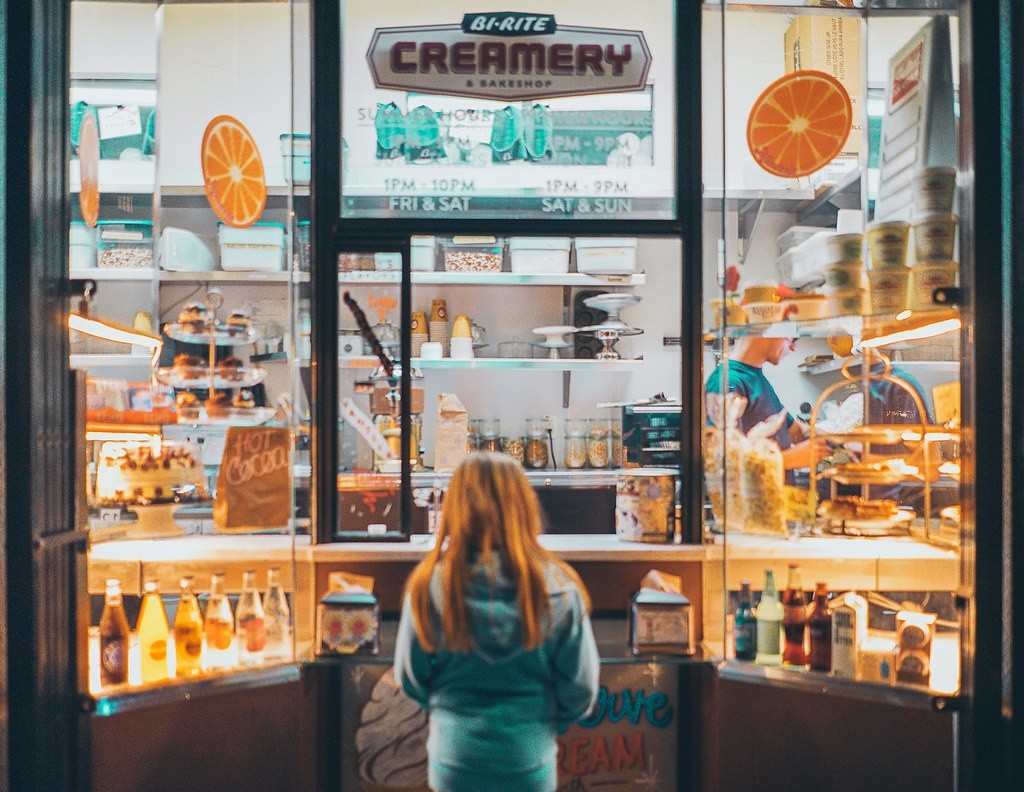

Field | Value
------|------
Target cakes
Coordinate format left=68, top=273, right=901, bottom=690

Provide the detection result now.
left=93, top=443, right=210, bottom=502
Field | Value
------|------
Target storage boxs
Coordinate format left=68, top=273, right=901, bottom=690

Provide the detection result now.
left=64, top=131, right=640, bottom=275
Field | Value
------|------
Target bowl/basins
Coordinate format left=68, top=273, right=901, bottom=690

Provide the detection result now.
left=367, top=524, right=387, bottom=536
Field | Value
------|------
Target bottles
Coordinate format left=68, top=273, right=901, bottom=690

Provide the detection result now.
left=755, top=568, right=784, bottom=665
left=611, top=418, right=622, bottom=469
left=99, top=579, right=131, bottom=689
left=173, top=575, right=204, bottom=678
left=564, top=418, right=587, bottom=469
left=807, top=581, right=833, bottom=673
left=466, top=416, right=550, bottom=468
left=262, top=566, right=291, bottom=664
left=450, top=314, right=475, bottom=359
left=734, top=580, right=756, bottom=661
left=588, top=419, right=610, bottom=468
left=205, top=570, right=235, bottom=668
left=135, top=577, right=169, bottom=681
left=782, top=563, right=808, bottom=666
left=235, top=567, right=265, bottom=667
left=470, top=317, right=487, bottom=343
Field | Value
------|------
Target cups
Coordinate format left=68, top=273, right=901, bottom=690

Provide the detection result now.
left=409, top=299, right=450, bottom=357
left=710, top=162, right=958, bottom=329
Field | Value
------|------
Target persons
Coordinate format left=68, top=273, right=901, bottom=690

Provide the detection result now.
left=394, top=449, right=601, bottom=792
left=852, top=350, right=950, bottom=519
left=706, top=326, right=862, bottom=611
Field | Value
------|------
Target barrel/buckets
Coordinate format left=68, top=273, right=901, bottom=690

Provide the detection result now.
left=614, top=468, right=681, bottom=544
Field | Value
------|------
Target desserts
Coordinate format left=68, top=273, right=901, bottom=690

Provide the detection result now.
left=173, top=305, right=255, bottom=421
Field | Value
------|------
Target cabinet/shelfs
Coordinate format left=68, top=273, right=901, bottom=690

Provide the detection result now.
left=702, top=166, right=964, bottom=695
left=66, top=74, right=648, bottom=539
